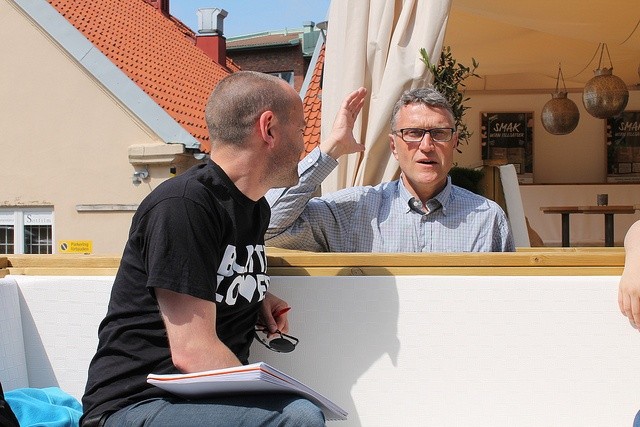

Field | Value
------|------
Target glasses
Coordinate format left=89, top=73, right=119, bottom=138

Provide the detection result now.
left=392, top=127, right=456, bottom=143
left=254, top=323, right=300, bottom=353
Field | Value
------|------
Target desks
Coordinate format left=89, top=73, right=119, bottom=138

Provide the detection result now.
left=539, top=205, right=635, bottom=247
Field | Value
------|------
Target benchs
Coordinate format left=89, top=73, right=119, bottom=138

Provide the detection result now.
left=0, top=273, right=640, bottom=427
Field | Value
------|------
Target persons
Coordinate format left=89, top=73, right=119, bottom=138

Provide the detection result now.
left=618, top=220, right=640, bottom=333
left=79, top=71, right=326, bottom=427
left=262, top=86, right=517, bottom=252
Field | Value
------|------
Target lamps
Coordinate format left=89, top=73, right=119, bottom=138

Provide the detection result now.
left=540, top=63, right=580, bottom=135
left=582, top=43, right=629, bottom=119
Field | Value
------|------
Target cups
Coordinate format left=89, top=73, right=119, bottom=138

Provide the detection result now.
left=597, top=194, right=609, bottom=207
